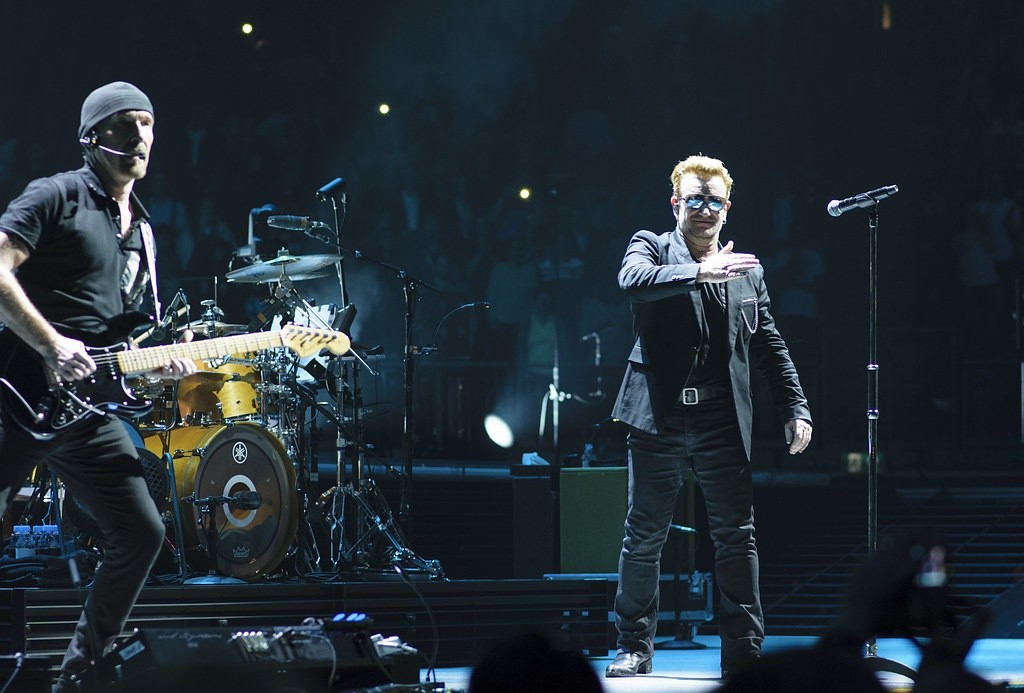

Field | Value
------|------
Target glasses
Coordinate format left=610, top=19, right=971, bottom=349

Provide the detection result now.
left=677, top=193, right=728, bottom=211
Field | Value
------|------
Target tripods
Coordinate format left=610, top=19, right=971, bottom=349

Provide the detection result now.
left=278, top=262, right=448, bottom=581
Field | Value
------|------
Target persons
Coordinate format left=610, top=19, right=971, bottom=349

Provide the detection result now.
left=605, top=156, right=812, bottom=677
left=0, top=81, right=194, bottom=693
left=467, top=629, right=603, bottom=693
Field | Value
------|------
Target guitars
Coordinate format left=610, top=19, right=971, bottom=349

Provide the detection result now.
left=0, top=306, right=352, bottom=497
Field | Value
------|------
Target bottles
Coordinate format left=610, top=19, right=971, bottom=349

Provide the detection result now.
left=582, top=444, right=597, bottom=467
left=9, top=525, right=61, bottom=560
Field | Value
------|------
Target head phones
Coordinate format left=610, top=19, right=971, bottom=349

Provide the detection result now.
left=79, top=129, right=98, bottom=149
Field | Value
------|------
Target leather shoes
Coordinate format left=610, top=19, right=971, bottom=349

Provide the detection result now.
left=723, top=652, right=761, bottom=681
left=606, top=649, right=653, bottom=678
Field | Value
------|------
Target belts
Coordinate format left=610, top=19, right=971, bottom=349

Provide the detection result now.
left=673, top=379, right=735, bottom=405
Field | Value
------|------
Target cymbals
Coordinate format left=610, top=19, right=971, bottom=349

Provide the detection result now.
left=225, top=252, right=345, bottom=283
left=169, top=321, right=250, bottom=338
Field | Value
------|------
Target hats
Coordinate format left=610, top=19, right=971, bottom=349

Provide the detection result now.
left=79, top=81, right=156, bottom=149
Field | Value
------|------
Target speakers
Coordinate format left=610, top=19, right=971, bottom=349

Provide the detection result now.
left=559, top=466, right=699, bottom=571
left=59, top=624, right=386, bottom=693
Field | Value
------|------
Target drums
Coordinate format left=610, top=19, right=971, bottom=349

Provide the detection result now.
left=138, top=353, right=302, bottom=583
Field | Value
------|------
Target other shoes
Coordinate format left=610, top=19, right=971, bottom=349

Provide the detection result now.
left=53, top=672, right=118, bottom=693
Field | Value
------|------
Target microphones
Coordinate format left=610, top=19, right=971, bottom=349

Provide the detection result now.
left=265, top=215, right=328, bottom=231
left=231, top=491, right=262, bottom=511
left=151, top=288, right=182, bottom=341
left=315, top=177, right=346, bottom=202
left=99, top=146, right=137, bottom=158
left=828, top=185, right=899, bottom=216
left=246, top=289, right=299, bottom=334
